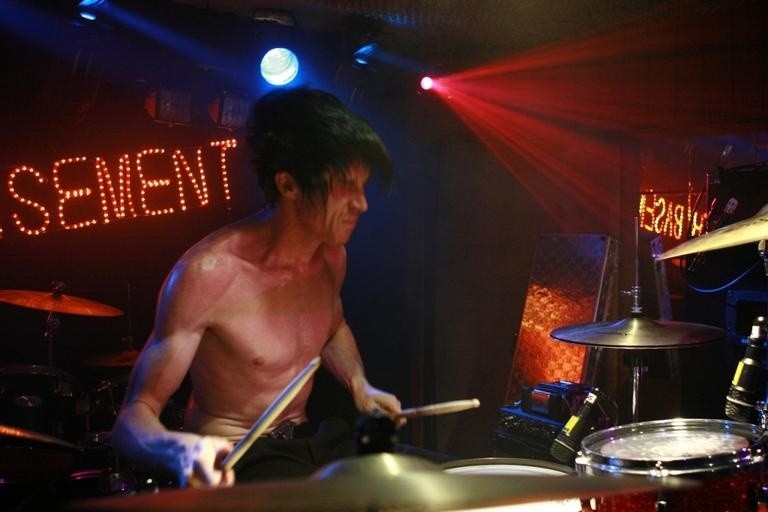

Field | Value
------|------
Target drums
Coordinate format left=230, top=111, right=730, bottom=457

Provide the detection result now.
left=439, top=457, right=574, bottom=511
left=575, top=418, right=766, bottom=512
left=0, top=365, right=69, bottom=434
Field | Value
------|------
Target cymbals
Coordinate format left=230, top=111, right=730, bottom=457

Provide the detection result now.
left=71, top=453, right=664, bottom=510
left=0, top=280, right=124, bottom=318
left=84, top=349, right=141, bottom=366
left=0, top=425, right=75, bottom=448
left=654, top=204, right=768, bottom=262
left=549, top=317, right=726, bottom=350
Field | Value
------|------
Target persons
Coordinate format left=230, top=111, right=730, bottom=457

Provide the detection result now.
left=108, top=83, right=465, bottom=491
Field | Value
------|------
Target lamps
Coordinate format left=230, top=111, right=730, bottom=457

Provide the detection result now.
left=252, top=7, right=300, bottom=88
